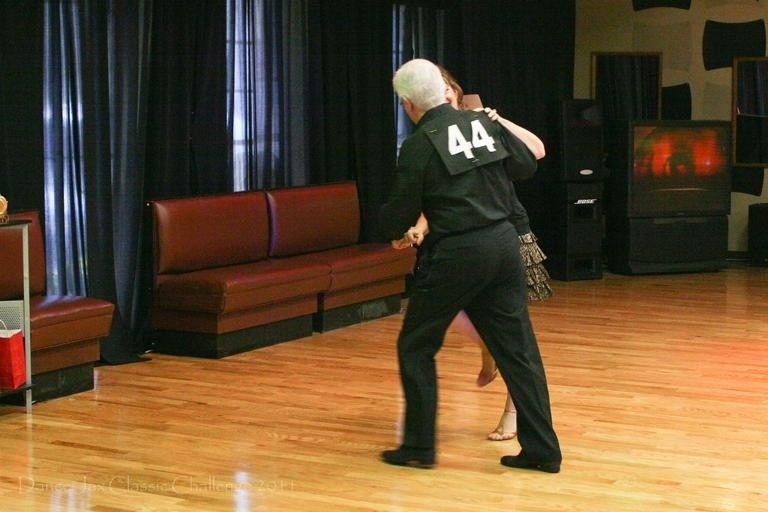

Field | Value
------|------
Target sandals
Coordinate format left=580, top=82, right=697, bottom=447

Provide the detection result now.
left=489, top=410, right=518, bottom=441
left=477, top=350, right=499, bottom=387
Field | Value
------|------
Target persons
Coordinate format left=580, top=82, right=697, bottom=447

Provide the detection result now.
left=371, top=58, right=562, bottom=472
left=392, top=65, right=553, bottom=442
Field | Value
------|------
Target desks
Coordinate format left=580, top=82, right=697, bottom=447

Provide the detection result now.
left=615, top=216, right=728, bottom=275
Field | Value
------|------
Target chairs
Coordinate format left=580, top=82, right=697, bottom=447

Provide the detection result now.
left=0, top=210, right=115, bottom=406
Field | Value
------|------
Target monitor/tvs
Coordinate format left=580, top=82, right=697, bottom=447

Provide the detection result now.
left=625, top=118, right=734, bottom=217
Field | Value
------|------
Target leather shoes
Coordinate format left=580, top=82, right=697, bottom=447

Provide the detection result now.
left=501, top=454, right=560, bottom=473
left=381, top=445, right=435, bottom=464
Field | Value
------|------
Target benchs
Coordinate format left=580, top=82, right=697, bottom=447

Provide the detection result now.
left=145, top=188, right=330, bottom=360
left=266, top=180, right=418, bottom=333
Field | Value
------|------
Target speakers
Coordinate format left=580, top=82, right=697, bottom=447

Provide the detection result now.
left=748, top=203, right=768, bottom=267
left=545, top=99, right=605, bottom=183
left=545, top=183, right=603, bottom=282
